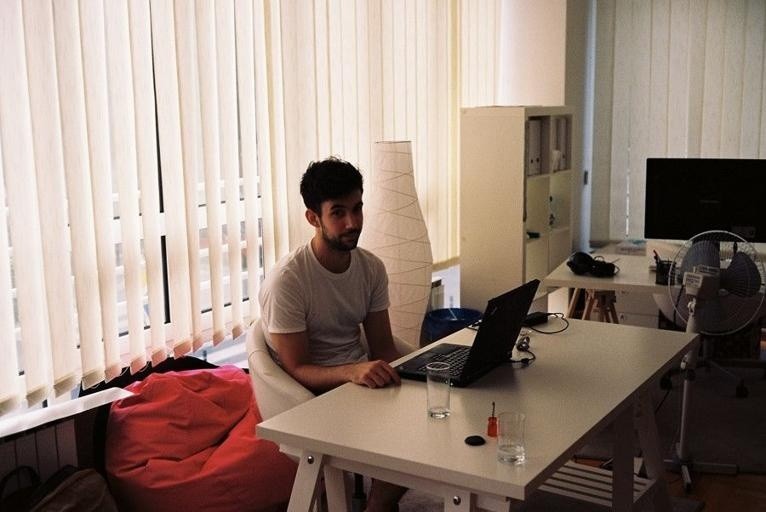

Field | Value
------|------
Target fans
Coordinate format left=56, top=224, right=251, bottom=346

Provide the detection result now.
left=652, top=228, right=764, bottom=490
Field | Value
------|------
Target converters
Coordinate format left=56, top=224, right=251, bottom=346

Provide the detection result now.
left=523, top=311, right=548, bottom=328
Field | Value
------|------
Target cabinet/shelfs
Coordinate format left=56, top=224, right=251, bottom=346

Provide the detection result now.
left=459, top=107, right=584, bottom=315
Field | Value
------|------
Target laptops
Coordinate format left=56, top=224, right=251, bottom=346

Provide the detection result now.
left=393, top=278, right=541, bottom=388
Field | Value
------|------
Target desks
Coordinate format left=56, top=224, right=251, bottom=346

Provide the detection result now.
left=542, top=252, right=765, bottom=322
left=254, top=310, right=701, bottom=511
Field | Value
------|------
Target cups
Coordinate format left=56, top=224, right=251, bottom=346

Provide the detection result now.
left=426, top=363, right=451, bottom=418
left=496, top=411, right=527, bottom=467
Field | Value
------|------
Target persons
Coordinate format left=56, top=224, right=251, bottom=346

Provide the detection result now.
left=257, top=155, right=411, bottom=511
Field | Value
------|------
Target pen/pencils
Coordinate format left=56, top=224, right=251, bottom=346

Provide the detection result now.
left=652, top=249, right=662, bottom=264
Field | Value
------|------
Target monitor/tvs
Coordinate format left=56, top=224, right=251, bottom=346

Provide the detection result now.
left=643, top=158, right=766, bottom=252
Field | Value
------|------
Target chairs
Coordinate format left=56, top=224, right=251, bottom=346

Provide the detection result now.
left=650, top=290, right=764, bottom=400
left=245, top=316, right=425, bottom=511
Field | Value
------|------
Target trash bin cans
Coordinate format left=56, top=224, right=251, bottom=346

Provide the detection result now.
left=426, top=307, right=483, bottom=346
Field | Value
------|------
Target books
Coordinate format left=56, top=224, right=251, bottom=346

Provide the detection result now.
left=526, top=117, right=569, bottom=176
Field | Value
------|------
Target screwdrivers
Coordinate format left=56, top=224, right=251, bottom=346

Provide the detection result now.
left=487, top=401, right=498, bottom=438
